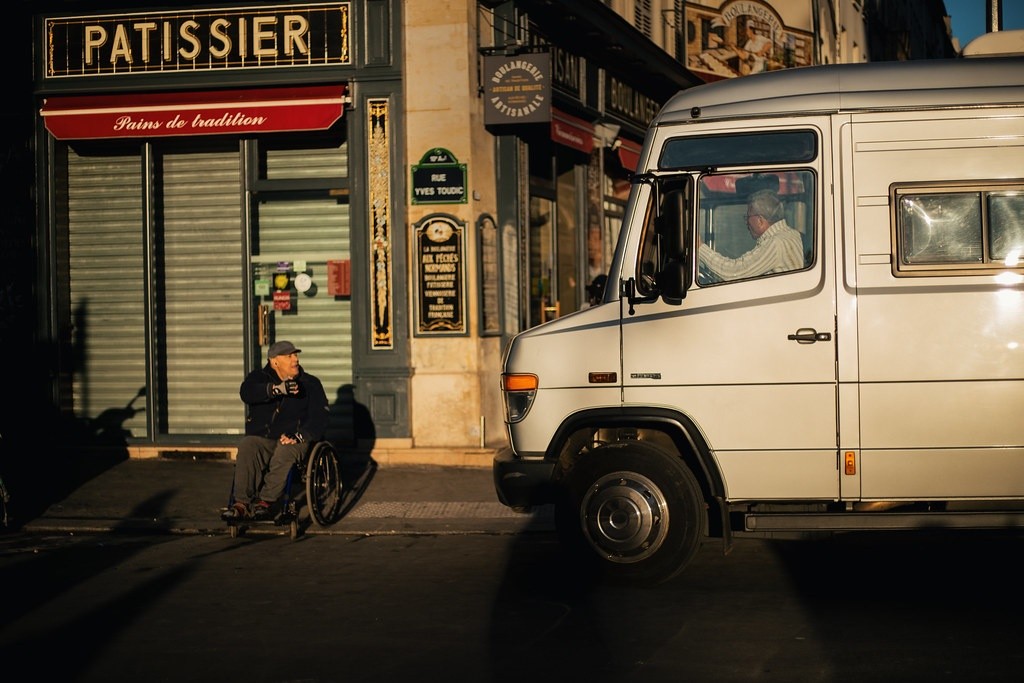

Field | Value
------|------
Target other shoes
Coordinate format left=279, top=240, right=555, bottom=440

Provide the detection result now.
left=219, top=504, right=268, bottom=523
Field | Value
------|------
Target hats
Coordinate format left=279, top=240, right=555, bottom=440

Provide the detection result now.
left=268, top=341, right=301, bottom=358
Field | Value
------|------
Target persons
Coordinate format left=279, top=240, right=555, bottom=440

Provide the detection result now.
left=699, top=195, right=805, bottom=282
left=220, top=341, right=331, bottom=522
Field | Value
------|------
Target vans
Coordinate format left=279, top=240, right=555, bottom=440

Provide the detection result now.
left=493, top=30, right=1024, bottom=589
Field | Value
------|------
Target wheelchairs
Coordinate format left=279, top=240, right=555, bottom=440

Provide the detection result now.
left=227, top=440, right=342, bottom=543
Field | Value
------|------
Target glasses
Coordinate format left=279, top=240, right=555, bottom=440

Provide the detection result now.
left=743, top=214, right=758, bottom=220
